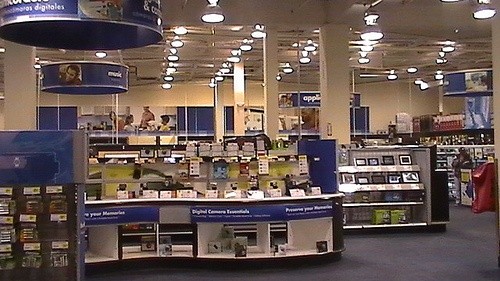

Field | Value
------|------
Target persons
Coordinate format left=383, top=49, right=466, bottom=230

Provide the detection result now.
left=110, top=107, right=172, bottom=132
left=452, top=151, right=471, bottom=206
left=63, top=64, right=81, bottom=83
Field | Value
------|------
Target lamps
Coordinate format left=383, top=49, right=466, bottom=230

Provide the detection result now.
left=472, top=0, right=498, bottom=19
left=200, top=0, right=224, bottom=23
left=359, top=24, right=384, bottom=40
left=363, top=4, right=381, bottom=25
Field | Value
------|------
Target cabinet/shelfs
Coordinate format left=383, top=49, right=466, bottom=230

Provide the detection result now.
left=82, top=142, right=452, bottom=234
left=434, top=144, right=495, bottom=200
left=83, top=191, right=345, bottom=275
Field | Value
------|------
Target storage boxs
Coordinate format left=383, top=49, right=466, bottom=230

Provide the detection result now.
left=354, top=191, right=409, bottom=224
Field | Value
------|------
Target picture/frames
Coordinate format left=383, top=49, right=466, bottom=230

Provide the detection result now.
left=341, top=154, right=420, bottom=183
